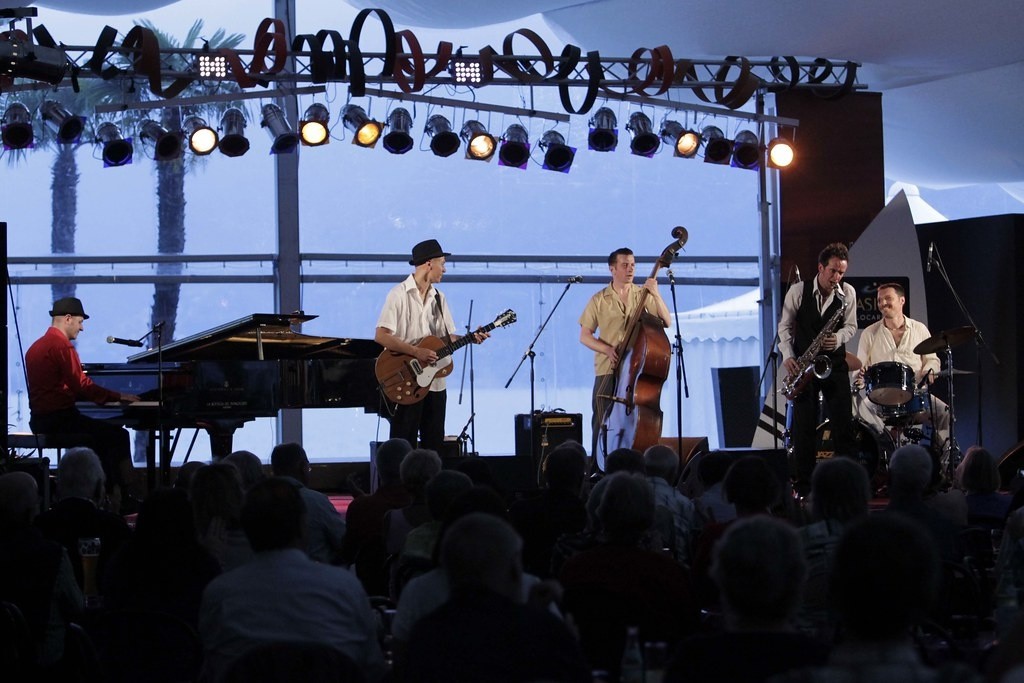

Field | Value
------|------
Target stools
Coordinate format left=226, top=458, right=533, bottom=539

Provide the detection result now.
left=885, top=417, right=922, bottom=449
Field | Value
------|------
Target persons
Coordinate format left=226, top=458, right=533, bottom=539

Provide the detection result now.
left=578, top=247, right=671, bottom=475
left=25, top=297, right=141, bottom=504
left=375, top=240, right=491, bottom=460
left=778, top=244, right=857, bottom=509
left=0, top=436, right=1024, bottom=683
left=851, top=282, right=953, bottom=499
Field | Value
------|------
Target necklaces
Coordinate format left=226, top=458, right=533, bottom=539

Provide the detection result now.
left=885, top=321, right=906, bottom=330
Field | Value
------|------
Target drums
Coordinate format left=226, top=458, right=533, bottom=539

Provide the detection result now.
left=863, top=361, right=916, bottom=406
left=876, top=389, right=930, bottom=424
left=816, top=417, right=898, bottom=499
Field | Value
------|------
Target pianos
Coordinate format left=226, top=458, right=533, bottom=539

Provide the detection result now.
left=73, top=309, right=391, bottom=499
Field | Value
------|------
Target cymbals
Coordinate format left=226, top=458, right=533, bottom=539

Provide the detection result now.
left=930, top=367, right=975, bottom=375
left=845, top=349, right=862, bottom=371
left=913, top=325, right=983, bottom=355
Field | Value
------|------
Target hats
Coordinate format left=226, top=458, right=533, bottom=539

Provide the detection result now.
left=409, top=239, right=451, bottom=265
left=49, top=298, right=89, bottom=319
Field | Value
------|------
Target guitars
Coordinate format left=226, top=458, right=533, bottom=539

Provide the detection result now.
left=374, top=309, right=517, bottom=405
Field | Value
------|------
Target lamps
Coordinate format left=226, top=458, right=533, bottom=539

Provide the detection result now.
left=0, top=17, right=68, bottom=86
left=625, top=101, right=661, bottom=159
left=660, top=108, right=702, bottom=160
left=419, top=103, right=461, bottom=158
left=194, top=52, right=228, bottom=81
left=766, top=125, right=797, bottom=169
left=383, top=98, right=417, bottom=155
left=136, top=118, right=185, bottom=162
left=587, top=98, right=619, bottom=153
left=259, top=96, right=303, bottom=155
left=460, top=108, right=499, bottom=163
left=697, top=114, right=736, bottom=166
left=91, top=112, right=133, bottom=167
left=1, top=92, right=35, bottom=151
left=450, top=57, right=487, bottom=88
left=37, top=99, right=88, bottom=145
left=217, top=101, right=250, bottom=157
left=538, top=120, right=577, bottom=175
left=729, top=118, right=766, bottom=172
left=498, top=114, right=531, bottom=170
left=181, top=114, right=219, bottom=156
left=329, top=93, right=385, bottom=149
left=299, top=93, right=332, bottom=147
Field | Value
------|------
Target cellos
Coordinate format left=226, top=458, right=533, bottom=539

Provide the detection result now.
left=596, top=227, right=690, bottom=472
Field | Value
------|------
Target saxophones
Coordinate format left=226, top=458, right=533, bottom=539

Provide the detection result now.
left=779, top=282, right=849, bottom=401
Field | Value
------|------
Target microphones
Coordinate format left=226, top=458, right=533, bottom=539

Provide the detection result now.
left=107, top=336, right=143, bottom=347
left=793, top=264, right=801, bottom=284
left=917, top=369, right=931, bottom=389
left=927, top=242, right=933, bottom=272
left=568, top=275, right=583, bottom=284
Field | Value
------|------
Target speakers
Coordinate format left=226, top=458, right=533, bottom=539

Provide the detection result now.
left=658, top=367, right=798, bottom=525
left=441, top=436, right=465, bottom=460
left=514, top=412, right=583, bottom=493
left=995, top=441, right=1024, bottom=493
left=9, top=456, right=52, bottom=511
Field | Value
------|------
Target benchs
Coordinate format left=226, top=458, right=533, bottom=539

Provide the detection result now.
left=9, top=433, right=64, bottom=463
